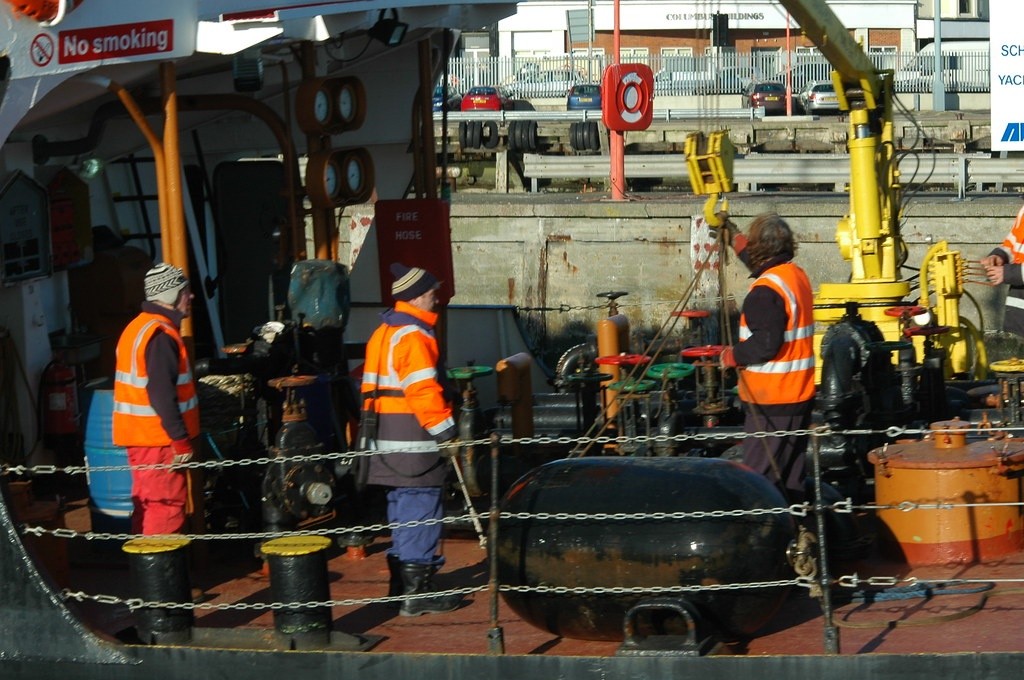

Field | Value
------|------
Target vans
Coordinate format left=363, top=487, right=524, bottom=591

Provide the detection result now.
left=894, top=40, right=989, bottom=90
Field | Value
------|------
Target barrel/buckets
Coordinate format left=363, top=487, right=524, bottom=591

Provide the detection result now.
left=83, top=388, right=140, bottom=518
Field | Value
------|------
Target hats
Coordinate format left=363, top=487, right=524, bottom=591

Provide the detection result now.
left=389, top=262, right=441, bottom=302
left=144, top=263, right=190, bottom=305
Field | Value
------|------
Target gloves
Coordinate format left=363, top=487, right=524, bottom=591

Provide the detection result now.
left=438, top=436, right=463, bottom=458
left=708, top=211, right=750, bottom=255
left=171, top=437, right=194, bottom=465
left=718, top=348, right=737, bottom=371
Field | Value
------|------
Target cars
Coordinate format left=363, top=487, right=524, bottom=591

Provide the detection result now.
left=797, top=80, right=841, bottom=113
left=461, top=86, right=515, bottom=111
left=741, top=82, right=787, bottom=113
left=500, top=69, right=587, bottom=96
left=432, top=82, right=461, bottom=112
left=566, top=84, right=601, bottom=111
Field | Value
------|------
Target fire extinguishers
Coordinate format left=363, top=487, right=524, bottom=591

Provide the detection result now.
left=38, top=354, right=78, bottom=440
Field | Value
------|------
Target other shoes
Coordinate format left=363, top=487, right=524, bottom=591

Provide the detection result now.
left=190, top=588, right=205, bottom=603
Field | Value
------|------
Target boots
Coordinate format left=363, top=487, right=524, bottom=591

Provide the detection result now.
left=387, top=555, right=403, bottom=605
left=400, top=556, right=463, bottom=616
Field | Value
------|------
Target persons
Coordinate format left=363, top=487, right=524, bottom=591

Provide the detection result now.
left=113, top=263, right=205, bottom=606
left=359, top=266, right=463, bottom=617
left=708, top=213, right=816, bottom=503
left=980, top=203, right=1024, bottom=336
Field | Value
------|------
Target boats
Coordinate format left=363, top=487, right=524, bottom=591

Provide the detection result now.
left=1, top=0, right=1024, bottom=678
